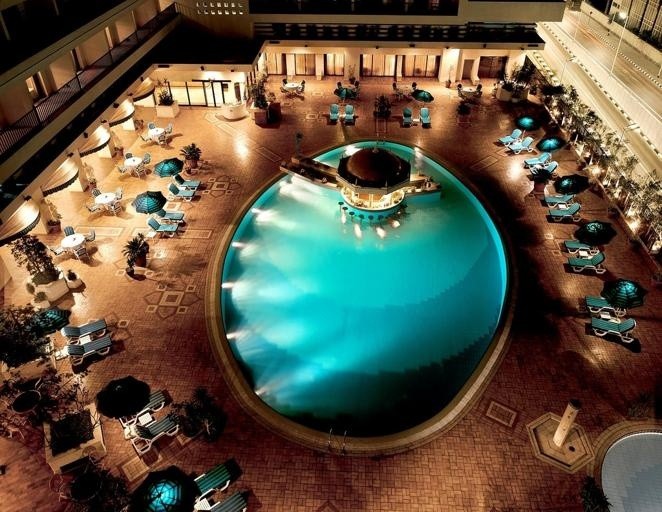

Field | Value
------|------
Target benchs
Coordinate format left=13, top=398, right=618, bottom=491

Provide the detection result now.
left=185, top=463, right=250, bottom=511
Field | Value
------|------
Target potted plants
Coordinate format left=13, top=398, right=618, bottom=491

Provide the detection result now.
left=25, top=283, right=51, bottom=310
left=64, top=268, right=84, bottom=291
left=153, top=77, right=181, bottom=118
left=455, top=102, right=472, bottom=126
left=121, top=231, right=150, bottom=278
left=178, top=141, right=203, bottom=175
left=166, top=387, right=227, bottom=445
left=496, top=80, right=522, bottom=104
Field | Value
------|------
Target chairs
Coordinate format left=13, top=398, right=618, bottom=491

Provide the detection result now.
left=59, top=318, right=114, bottom=367
left=329, top=78, right=359, bottom=125
left=46, top=184, right=127, bottom=265
left=114, top=121, right=175, bottom=179
left=281, top=75, right=308, bottom=101
left=499, top=126, right=643, bottom=344
left=399, top=103, right=432, bottom=128
left=116, top=390, right=184, bottom=460
left=147, top=174, right=202, bottom=238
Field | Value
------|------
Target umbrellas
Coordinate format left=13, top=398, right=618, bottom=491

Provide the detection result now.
left=334, top=88, right=357, bottom=106
left=412, top=89, right=434, bottom=107
left=130, top=465, right=202, bottom=512
left=27, top=305, right=71, bottom=338
left=575, top=220, right=617, bottom=251
left=514, top=116, right=541, bottom=140
left=601, top=278, right=648, bottom=319
left=536, top=136, right=566, bottom=161
left=132, top=191, right=167, bottom=215
left=153, top=157, right=184, bottom=184
left=554, top=174, right=589, bottom=198
left=95, top=375, right=151, bottom=431
